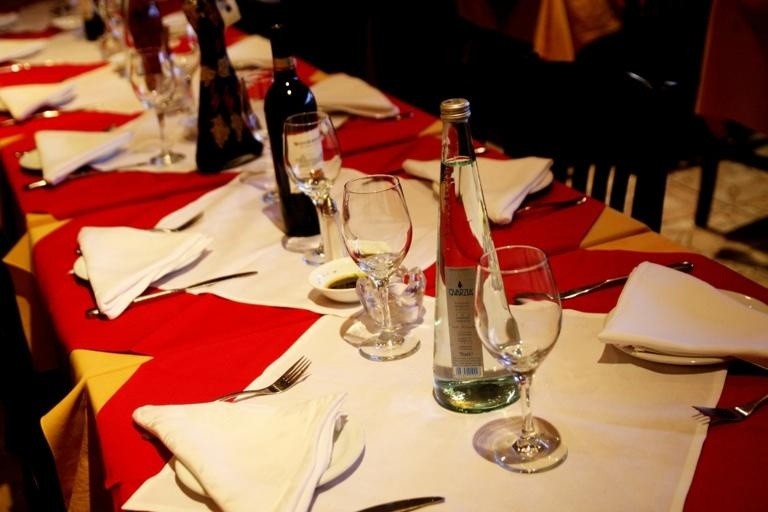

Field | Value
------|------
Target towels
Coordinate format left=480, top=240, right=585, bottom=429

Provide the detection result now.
left=0, top=9, right=767, bottom=511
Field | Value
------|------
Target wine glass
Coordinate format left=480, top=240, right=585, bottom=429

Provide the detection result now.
left=128, top=24, right=200, bottom=168
left=280, top=111, right=422, bottom=361
left=473, top=245, right=568, bottom=473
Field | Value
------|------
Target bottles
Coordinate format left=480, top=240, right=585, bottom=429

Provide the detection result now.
left=264, top=22, right=337, bottom=238
left=428, top=98, right=526, bottom=413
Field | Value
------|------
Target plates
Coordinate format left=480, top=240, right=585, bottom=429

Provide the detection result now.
left=18, top=146, right=56, bottom=171
left=605, top=292, right=768, bottom=370
left=175, top=412, right=367, bottom=498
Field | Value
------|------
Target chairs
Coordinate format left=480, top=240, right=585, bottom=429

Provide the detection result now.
left=551, top=70, right=717, bottom=237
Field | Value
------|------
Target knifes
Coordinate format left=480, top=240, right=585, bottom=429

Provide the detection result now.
left=358, top=496, right=446, bottom=512
left=559, top=259, right=693, bottom=300
left=86, top=271, right=258, bottom=317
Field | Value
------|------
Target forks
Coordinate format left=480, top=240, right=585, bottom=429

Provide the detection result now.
left=212, top=356, right=311, bottom=402
left=691, top=395, right=767, bottom=424
